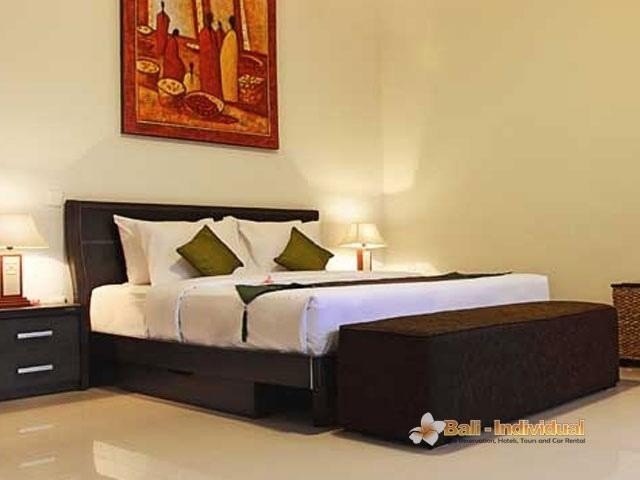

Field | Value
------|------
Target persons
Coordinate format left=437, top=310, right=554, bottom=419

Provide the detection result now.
left=163, top=28, right=186, bottom=85
left=220, top=17, right=241, bottom=104
left=196, top=11, right=222, bottom=102
left=183, top=62, right=204, bottom=93
left=155, top=2, right=171, bottom=56
left=216, top=20, right=227, bottom=52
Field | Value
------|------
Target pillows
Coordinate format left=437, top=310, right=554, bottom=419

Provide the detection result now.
left=138, top=223, right=253, bottom=286
left=111, top=211, right=217, bottom=282
left=179, top=223, right=244, bottom=277
left=241, top=220, right=320, bottom=273
left=278, top=228, right=330, bottom=269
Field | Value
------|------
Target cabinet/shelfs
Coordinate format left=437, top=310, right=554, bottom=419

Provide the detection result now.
left=0, top=302, right=89, bottom=402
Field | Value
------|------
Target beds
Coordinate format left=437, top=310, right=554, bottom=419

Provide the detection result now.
left=64, top=197, right=548, bottom=430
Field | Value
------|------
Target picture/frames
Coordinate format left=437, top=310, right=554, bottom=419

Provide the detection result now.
left=118, top=0, right=280, bottom=152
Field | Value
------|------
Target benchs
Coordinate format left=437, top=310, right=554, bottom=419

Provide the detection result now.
left=333, top=299, right=622, bottom=451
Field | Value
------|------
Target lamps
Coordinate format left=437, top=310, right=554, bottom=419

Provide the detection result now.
left=340, top=221, right=386, bottom=271
left=0, top=213, right=62, bottom=312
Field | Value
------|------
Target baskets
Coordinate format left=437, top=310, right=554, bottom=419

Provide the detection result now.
left=611, top=287, right=640, bottom=361
